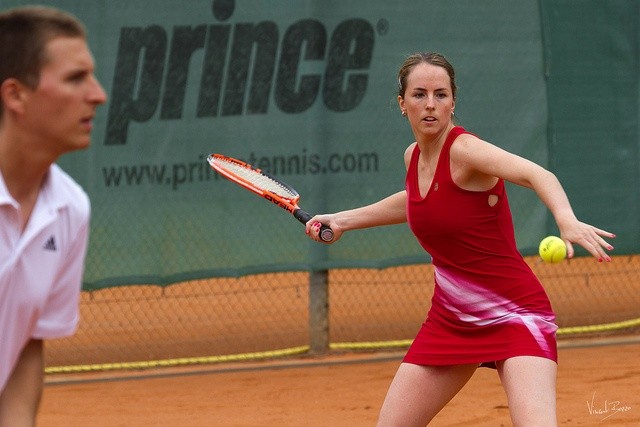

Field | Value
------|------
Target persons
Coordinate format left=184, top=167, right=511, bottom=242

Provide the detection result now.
left=303, top=50, right=619, bottom=427
left=0, top=8, right=107, bottom=426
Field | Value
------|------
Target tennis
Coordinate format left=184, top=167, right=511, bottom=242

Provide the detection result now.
left=538, top=235, right=567, bottom=264
